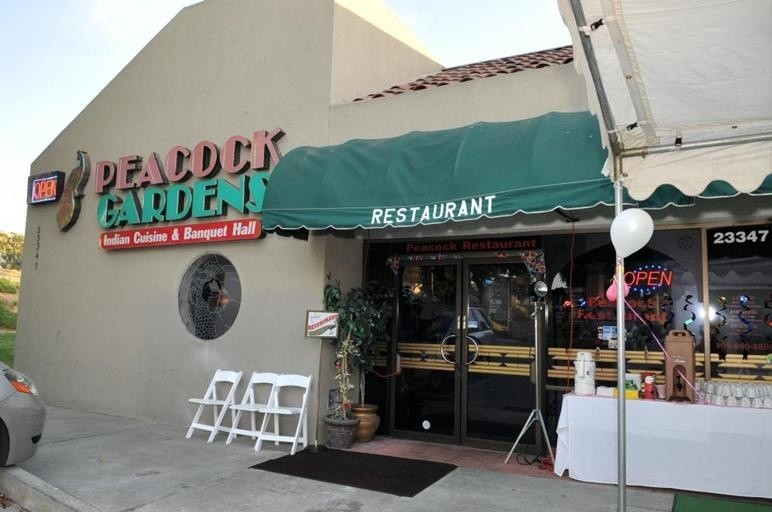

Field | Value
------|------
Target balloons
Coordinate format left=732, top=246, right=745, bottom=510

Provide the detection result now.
left=610, top=208, right=655, bottom=264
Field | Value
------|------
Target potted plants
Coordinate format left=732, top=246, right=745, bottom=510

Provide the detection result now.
left=321, top=272, right=392, bottom=450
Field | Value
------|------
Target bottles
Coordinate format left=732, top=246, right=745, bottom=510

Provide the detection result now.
left=573, top=351, right=596, bottom=397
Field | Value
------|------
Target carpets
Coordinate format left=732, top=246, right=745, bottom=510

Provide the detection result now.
left=248, top=443, right=459, bottom=499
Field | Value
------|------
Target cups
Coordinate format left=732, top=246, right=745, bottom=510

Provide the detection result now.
left=695, top=380, right=772, bottom=409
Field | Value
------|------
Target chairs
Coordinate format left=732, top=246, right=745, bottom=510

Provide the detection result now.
left=184, top=370, right=314, bottom=456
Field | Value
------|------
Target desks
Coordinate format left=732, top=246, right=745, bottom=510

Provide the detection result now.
left=554, top=389, right=772, bottom=500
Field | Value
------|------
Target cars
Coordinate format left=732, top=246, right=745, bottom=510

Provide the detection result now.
left=466, top=303, right=499, bottom=352
left=1, top=359, right=47, bottom=467
